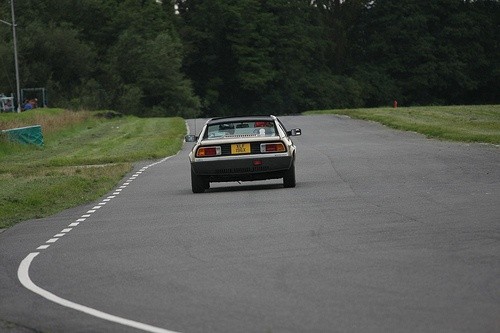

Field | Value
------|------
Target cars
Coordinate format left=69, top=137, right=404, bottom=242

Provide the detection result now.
left=184, top=114, right=302, bottom=194
